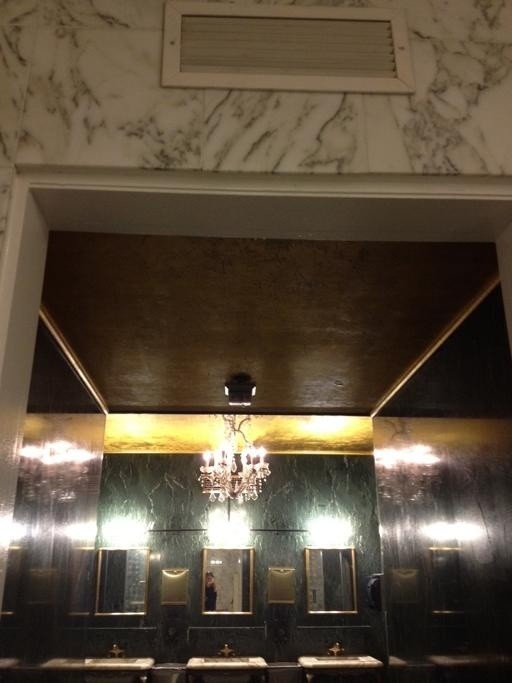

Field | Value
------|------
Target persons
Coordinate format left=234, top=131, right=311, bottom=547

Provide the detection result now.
left=204, top=570, right=218, bottom=611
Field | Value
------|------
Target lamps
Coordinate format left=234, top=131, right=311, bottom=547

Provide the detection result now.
left=223, top=374, right=256, bottom=405
left=197, top=414, right=271, bottom=504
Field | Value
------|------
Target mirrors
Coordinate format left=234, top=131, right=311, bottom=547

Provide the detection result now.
left=201, top=547, right=254, bottom=616
left=94, top=548, right=151, bottom=617
left=305, top=547, right=359, bottom=617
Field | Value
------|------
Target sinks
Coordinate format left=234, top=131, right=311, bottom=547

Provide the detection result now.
left=186, top=654, right=270, bottom=669
left=426, top=655, right=500, bottom=668
left=42, top=655, right=155, bottom=670
left=298, top=652, right=384, bottom=668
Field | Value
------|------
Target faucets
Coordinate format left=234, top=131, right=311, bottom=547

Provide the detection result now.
left=219, top=643, right=234, bottom=657
left=109, top=643, right=124, bottom=658
left=328, top=640, right=346, bottom=656
